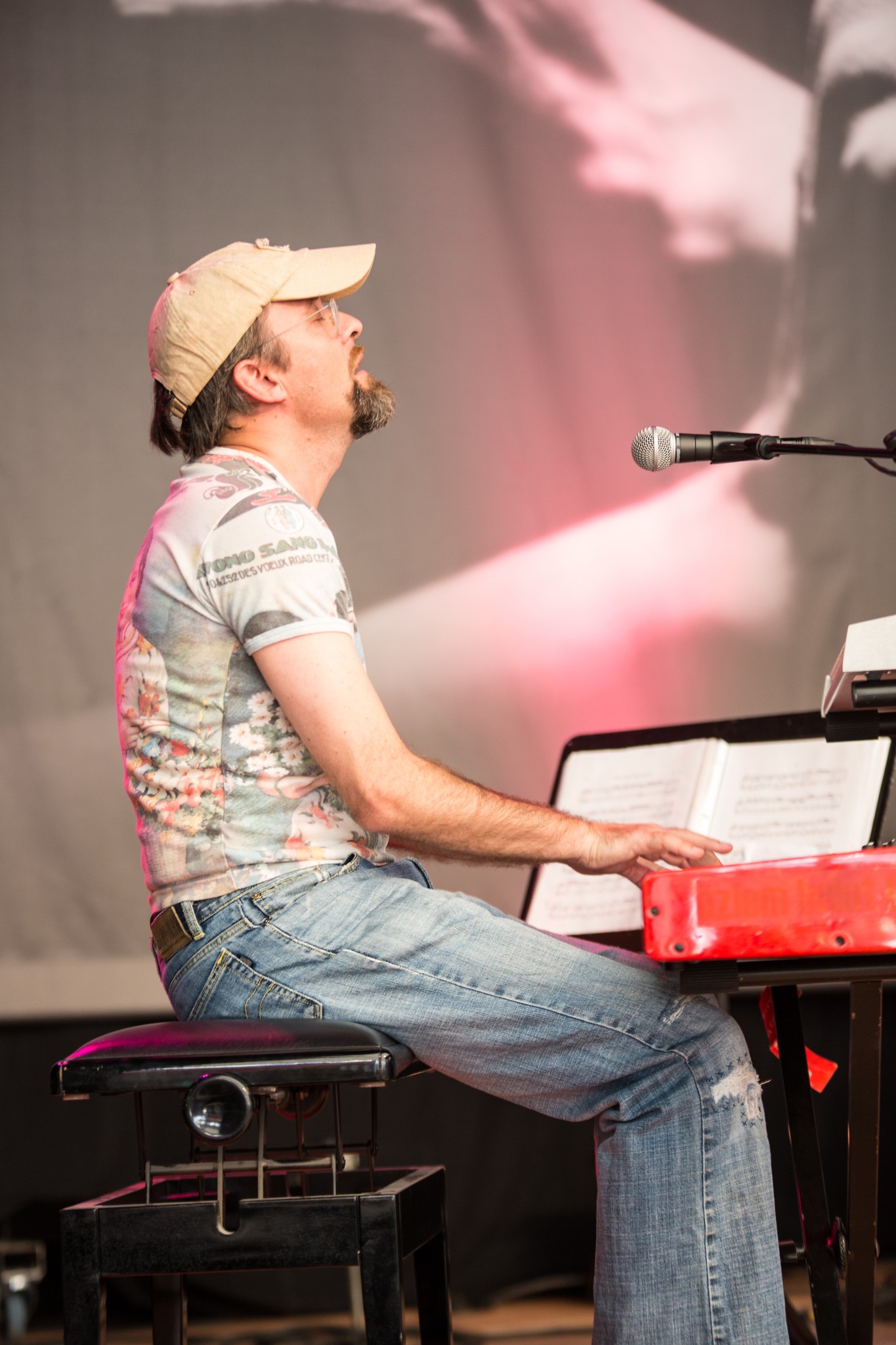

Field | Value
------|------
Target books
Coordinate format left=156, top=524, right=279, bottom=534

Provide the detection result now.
left=524, top=735, right=893, bottom=937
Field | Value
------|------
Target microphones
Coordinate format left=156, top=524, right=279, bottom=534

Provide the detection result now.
left=631, top=419, right=837, bottom=471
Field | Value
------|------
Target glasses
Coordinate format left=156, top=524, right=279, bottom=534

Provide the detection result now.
left=247, top=298, right=339, bottom=354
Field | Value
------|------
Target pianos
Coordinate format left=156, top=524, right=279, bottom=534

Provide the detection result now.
left=642, top=840, right=896, bottom=987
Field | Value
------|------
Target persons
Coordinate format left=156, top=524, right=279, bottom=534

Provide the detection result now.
left=0, top=0, right=896, bottom=960
left=112, top=234, right=795, bottom=1345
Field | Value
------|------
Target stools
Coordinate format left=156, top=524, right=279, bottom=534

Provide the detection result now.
left=54, top=1021, right=455, bottom=1345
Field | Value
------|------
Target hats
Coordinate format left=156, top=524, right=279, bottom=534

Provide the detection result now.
left=148, top=238, right=376, bottom=420
